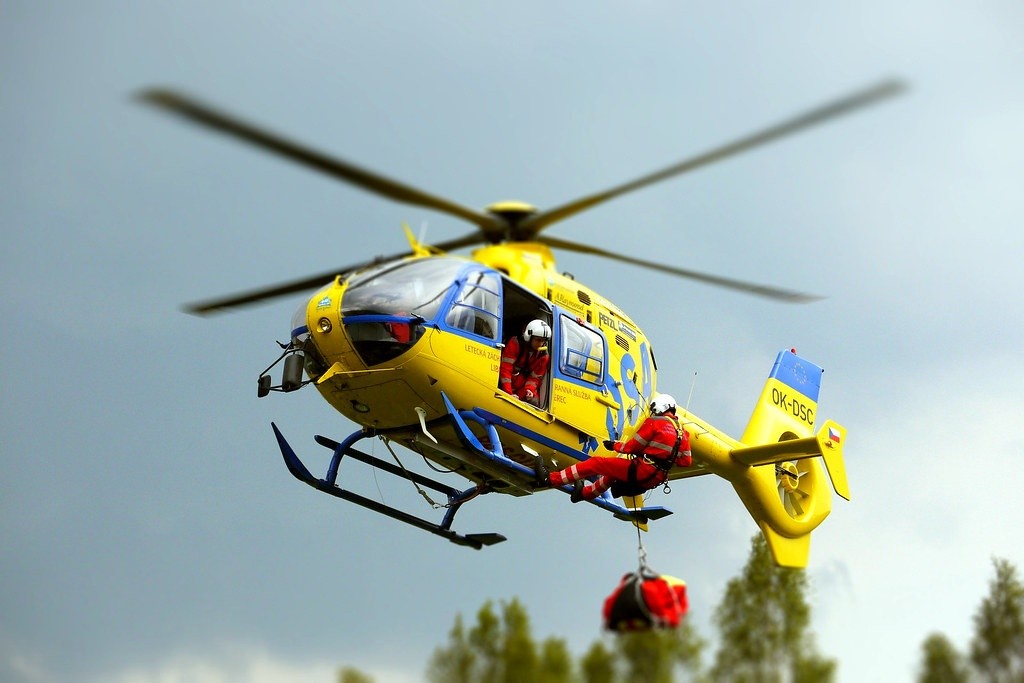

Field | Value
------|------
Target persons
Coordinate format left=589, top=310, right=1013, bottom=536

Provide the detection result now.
left=539, top=394, right=692, bottom=503
left=497, top=318, right=552, bottom=408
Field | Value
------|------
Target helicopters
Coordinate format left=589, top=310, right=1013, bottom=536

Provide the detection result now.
left=138, top=74, right=920, bottom=636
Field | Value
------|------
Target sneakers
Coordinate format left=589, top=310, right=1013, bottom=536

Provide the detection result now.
left=535, top=456, right=549, bottom=487
left=571, top=478, right=584, bottom=504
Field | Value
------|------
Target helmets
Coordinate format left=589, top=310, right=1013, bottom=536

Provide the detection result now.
left=649, top=393, right=676, bottom=415
left=524, top=318, right=551, bottom=342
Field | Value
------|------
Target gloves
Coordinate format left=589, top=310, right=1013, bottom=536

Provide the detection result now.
left=603, top=440, right=614, bottom=451
